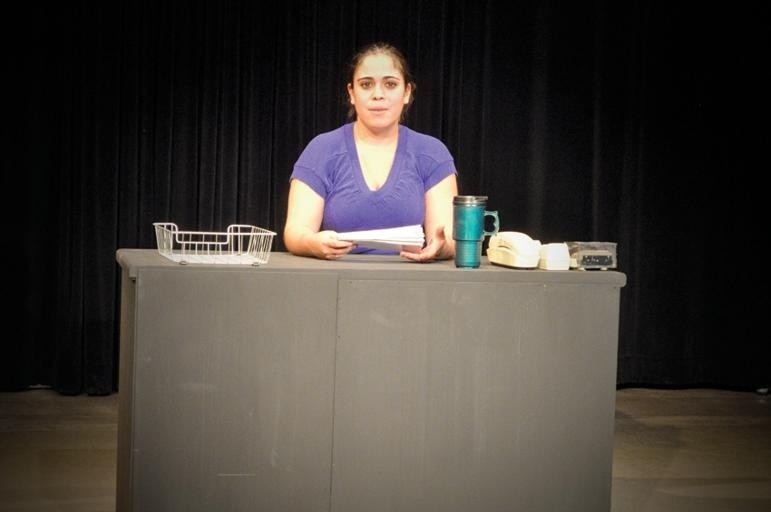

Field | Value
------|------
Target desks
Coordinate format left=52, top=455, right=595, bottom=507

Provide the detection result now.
left=116, top=248, right=627, bottom=512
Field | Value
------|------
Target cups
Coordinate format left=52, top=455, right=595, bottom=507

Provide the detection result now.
left=454, top=195, right=499, bottom=268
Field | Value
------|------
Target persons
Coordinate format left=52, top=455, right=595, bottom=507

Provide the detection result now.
left=284, top=43, right=458, bottom=264
